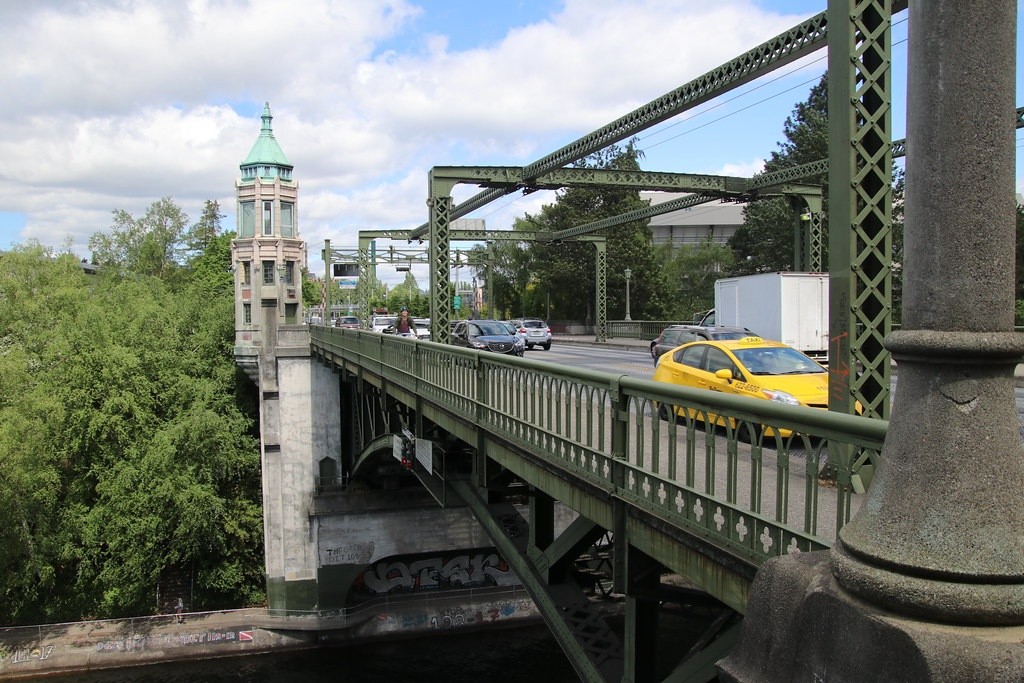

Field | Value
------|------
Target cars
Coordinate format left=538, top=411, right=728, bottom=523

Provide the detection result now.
left=496, top=320, right=526, bottom=351
left=649, top=338, right=865, bottom=446
left=651, top=337, right=660, bottom=358
left=329, top=315, right=467, bottom=340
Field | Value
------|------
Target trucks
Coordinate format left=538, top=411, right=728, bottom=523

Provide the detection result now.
left=698, top=271, right=829, bottom=363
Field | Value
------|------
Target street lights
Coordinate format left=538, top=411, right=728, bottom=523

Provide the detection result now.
left=624, top=267, right=634, bottom=322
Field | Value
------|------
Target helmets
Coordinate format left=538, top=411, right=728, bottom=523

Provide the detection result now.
left=399, top=306, right=409, bottom=312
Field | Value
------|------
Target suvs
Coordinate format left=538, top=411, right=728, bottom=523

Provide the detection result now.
left=654, top=324, right=763, bottom=370
left=450, top=319, right=524, bottom=356
left=505, top=318, right=552, bottom=351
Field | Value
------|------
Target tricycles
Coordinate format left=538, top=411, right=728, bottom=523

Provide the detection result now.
left=379, top=333, right=419, bottom=369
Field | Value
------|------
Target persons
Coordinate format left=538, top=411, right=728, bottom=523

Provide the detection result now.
left=392, top=306, right=419, bottom=340
left=175, top=597, right=183, bottom=621
